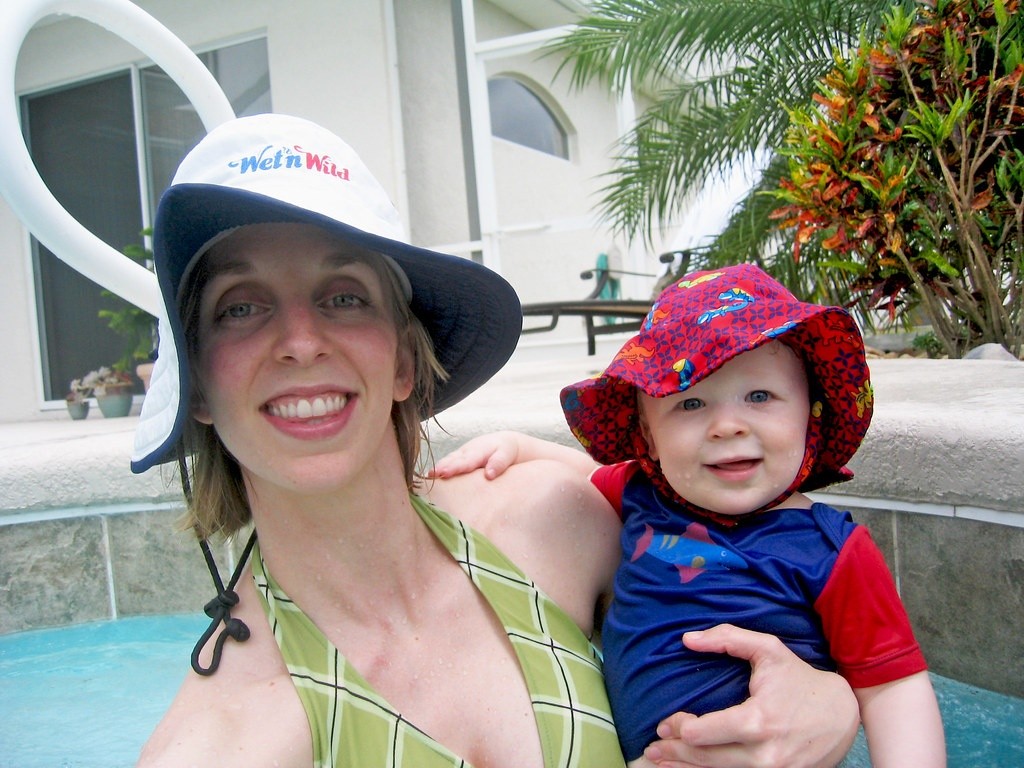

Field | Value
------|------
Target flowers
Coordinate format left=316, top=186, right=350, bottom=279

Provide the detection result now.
left=63, top=366, right=136, bottom=404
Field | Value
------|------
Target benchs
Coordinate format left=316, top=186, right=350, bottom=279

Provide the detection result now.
left=521, top=250, right=691, bottom=356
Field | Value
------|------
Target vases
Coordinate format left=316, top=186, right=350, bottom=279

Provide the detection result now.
left=67, top=397, right=90, bottom=420
left=95, top=383, right=129, bottom=418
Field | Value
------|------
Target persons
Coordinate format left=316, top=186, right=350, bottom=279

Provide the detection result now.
left=419, top=259, right=949, bottom=768
left=132, top=110, right=864, bottom=768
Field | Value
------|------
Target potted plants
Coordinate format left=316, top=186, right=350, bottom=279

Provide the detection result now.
left=98, top=226, right=153, bottom=396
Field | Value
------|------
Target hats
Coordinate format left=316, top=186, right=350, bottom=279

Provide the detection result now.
left=560, top=264, right=872, bottom=493
left=129, top=113, right=523, bottom=475
